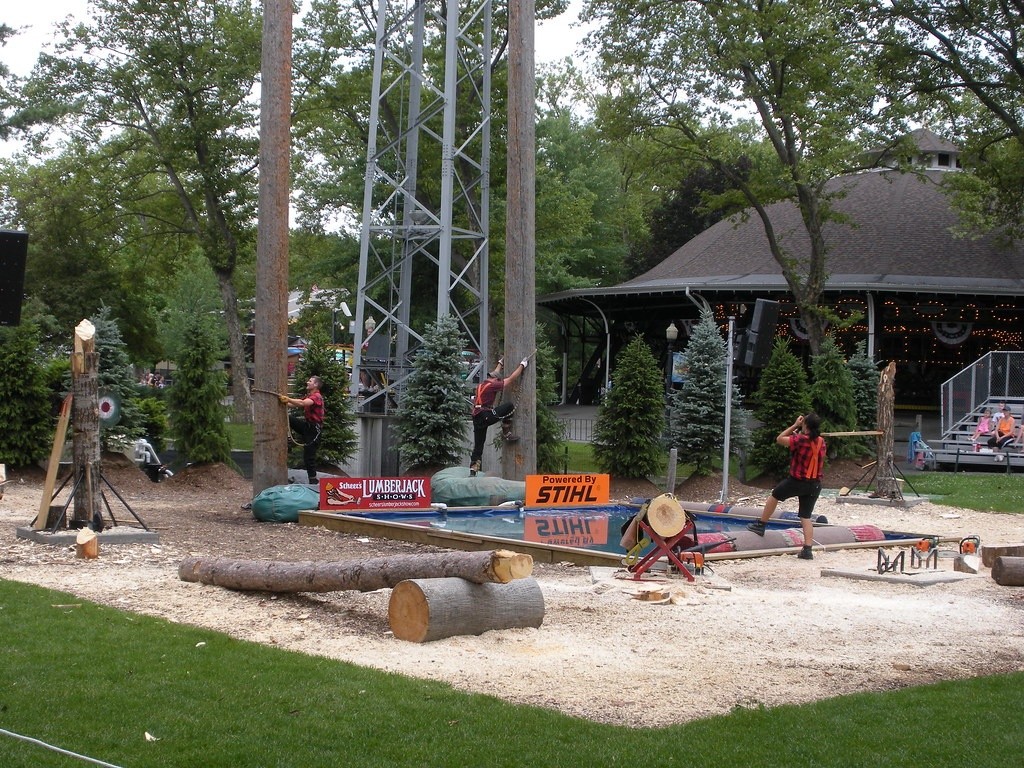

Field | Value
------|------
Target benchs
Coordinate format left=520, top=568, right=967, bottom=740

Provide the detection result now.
left=914, top=397, right=1023, bottom=473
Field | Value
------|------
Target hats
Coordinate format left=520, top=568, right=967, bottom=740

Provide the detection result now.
left=1002, top=406, right=1011, bottom=411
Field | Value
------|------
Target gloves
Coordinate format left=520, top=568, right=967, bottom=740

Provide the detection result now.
left=498, top=357, right=504, bottom=366
left=520, top=357, right=528, bottom=368
left=279, top=394, right=289, bottom=403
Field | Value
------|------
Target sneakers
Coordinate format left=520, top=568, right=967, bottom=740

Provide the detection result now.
left=798, top=545, right=813, bottom=559
left=745, top=521, right=765, bottom=537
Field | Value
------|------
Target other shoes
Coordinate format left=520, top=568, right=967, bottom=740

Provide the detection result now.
left=997, top=454, right=1004, bottom=461
left=994, top=456, right=998, bottom=462
left=309, top=478, right=319, bottom=484
left=971, top=438, right=976, bottom=442
left=502, top=432, right=521, bottom=442
left=470, top=468, right=477, bottom=476
left=967, top=438, right=971, bottom=441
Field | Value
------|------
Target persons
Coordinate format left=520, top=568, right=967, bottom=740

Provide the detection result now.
left=968, top=400, right=1015, bottom=462
left=147, top=371, right=165, bottom=388
left=746, top=413, right=826, bottom=559
left=470, top=356, right=528, bottom=475
left=279, top=376, right=324, bottom=486
